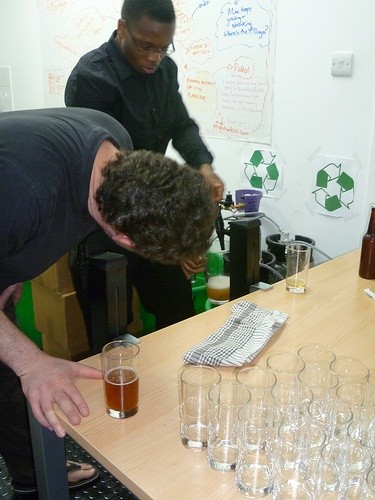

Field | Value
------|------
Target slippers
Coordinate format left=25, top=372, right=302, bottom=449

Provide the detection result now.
left=9, top=461, right=101, bottom=496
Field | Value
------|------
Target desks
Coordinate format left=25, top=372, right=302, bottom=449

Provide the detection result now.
left=27, top=247, right=375, bottom=500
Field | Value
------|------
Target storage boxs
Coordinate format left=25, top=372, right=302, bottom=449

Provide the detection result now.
left=31, top=253, right=91, bottom=363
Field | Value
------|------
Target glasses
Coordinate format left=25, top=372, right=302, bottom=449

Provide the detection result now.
left=126, top=22, right=175, bottom=56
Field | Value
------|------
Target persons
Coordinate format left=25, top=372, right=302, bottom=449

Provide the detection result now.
left=0, top=107, right=219, bottom=495
left=65, top=0, right=224, bottom=356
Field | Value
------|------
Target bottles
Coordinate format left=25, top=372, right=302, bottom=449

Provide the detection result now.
left=357, top=207, right=375, bottom=279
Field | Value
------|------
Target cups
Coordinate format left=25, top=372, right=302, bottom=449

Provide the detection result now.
left=285, top=244, right=311, bottom=294
left=179, top=365, right=222, bottom=450
left=207, top=273, right=230, bottom=302
left=206, top=345, right=375, bottom=500
left=102, top=341, right=140, bottom=419
